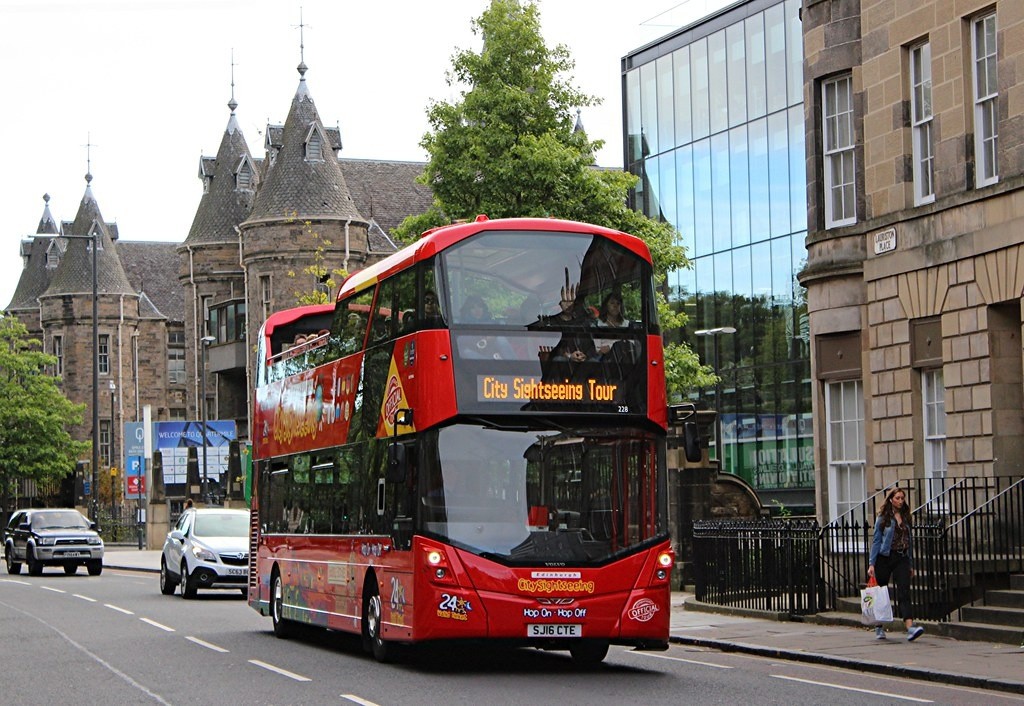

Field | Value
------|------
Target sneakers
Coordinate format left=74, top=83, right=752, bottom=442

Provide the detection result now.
left=907, top=626, right=924, bottom=641
left=875, top=627, right=886, bottom=639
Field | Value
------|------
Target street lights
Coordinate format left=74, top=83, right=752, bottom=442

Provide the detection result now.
left=200, top=334, right=216, bottom=502
left=76, top=458, right=93, bottom=520
left=39, top=232, right=97, bottom=528
left=693, top=325, right=739, bottom=473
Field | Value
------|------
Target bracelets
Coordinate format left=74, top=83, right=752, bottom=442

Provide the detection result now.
left=567, top=353, right=571, bottom=360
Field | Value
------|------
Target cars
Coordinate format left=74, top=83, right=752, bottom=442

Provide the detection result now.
left=159, top=507, right=274, bottom=599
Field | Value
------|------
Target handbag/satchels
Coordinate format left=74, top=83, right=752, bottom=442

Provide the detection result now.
left=860, top=576, right=894, bottom=625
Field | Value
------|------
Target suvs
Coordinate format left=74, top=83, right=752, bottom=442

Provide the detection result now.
left=3, top=508, right=104, bottom=575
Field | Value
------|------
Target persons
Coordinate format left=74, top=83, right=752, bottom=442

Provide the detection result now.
left=591, top=293, right=632, bottom=355
left=457, top=294, right=519, bottom=360
left=550, top=285, right=590, bottom=362
left=349, top=308, right=415, bottom=341
left=289, top=329, right=330, bottom=357
left=422, top=291, right=446, bottom=329
left=860, top=487, right=924, bottom=642
left=185, top=498, right=194, bottom=510
left=520, top=298, right=540, bottom=321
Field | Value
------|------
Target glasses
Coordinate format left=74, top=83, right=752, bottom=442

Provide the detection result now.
left=311, top=342, right=320, bottom=347
left=424, top=297, right=436, bottom=305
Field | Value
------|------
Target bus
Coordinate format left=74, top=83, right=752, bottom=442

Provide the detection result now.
left=245, top=214, right=707, bottom=666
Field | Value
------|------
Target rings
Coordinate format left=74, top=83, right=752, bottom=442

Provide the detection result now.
left=867, top=569, right=870, bottom=573
